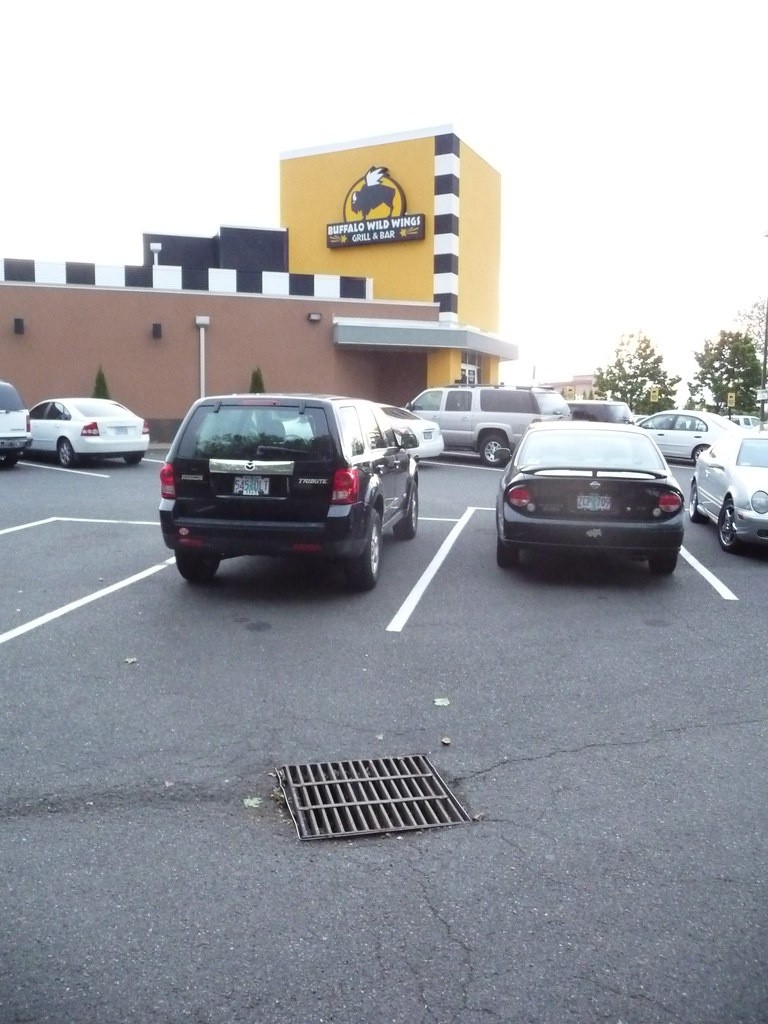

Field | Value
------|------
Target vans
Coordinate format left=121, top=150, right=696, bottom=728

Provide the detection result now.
left=400, top=383, right=572, bottom=466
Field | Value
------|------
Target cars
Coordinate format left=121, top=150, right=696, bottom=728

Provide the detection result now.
left=23, top=396, right=151, bottom=470
left=375, top=398, right=445, bottom=462
left=566, top=399, right=634, bottom=426
left=494, top=419, right=688, bottom=574
left=0, top=378, right=32, bottom=468
left=687, top=434, right=768, bottom=550
left=633, top=409, right=747, bottom=466
left=723, top=414, right=764, bottom=431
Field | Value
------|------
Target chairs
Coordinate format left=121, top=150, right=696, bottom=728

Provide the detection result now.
left=690, top=420, right=696, bottom=430
left=259, top=420, right=285, bottom=444
left=679, top=421, right=686, bottom=430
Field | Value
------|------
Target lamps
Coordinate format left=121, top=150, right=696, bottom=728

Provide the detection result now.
left=307, top=313, right=323, bottom=321
left=153, top=323, right=161, bottom=338
left=14, top=318, right=24, bottom=333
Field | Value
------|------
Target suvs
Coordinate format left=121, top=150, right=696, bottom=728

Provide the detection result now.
left=159, top=391, right=421, bottom=593
